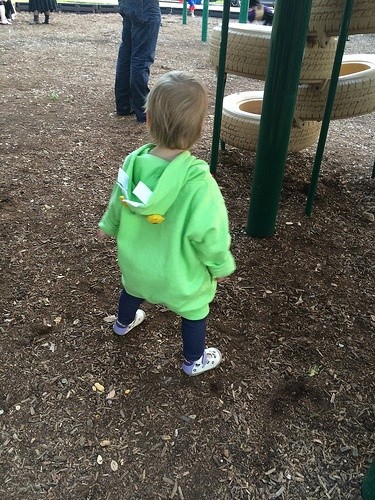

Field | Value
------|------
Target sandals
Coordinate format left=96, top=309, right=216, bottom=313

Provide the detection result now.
left=113, top=308, right=145, bottom=336
left=182, top=347, right=222, bottom=376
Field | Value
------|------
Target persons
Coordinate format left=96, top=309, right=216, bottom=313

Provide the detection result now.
left=114, top=0, right=161, bottom=123
left=97, top=70, right=237, bottom=378
left=0, top=0, right=16, bottom=25
left=29, top=0, right=58, bottom=25
left=231, top=0, right=241, bottom=7
left=248, top=0, right=273, bottom=26
left=187, top=0, right=195, bottom=17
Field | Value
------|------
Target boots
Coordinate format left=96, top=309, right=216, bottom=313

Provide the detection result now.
left=30, top=15, right=39, bottom=24
left=42, top=15, right=49, bottom=23
left=0, top=5, right=12, bottom=24
